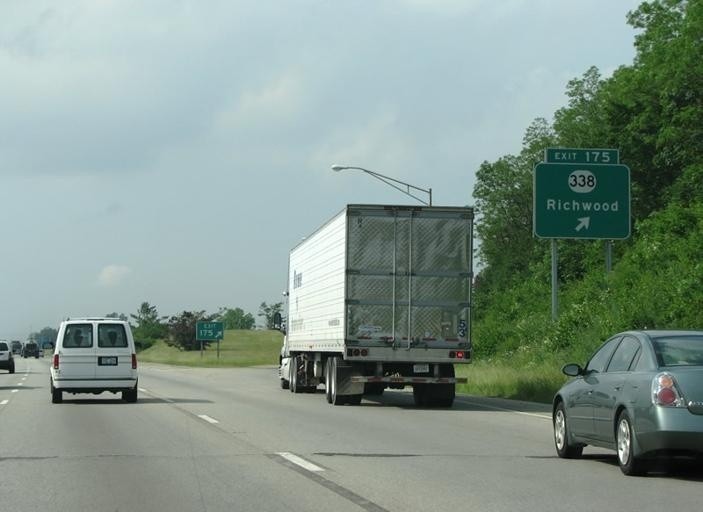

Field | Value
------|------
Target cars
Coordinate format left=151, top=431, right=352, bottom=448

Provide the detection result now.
left=39, top=348, right=45, bottom=358
left=551, top=327, right=703, bottom=481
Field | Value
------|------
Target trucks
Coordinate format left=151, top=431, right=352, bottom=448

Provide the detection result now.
left=9, top=336, right=41, bottom=358
left=277, top=203, right=476, bottom=409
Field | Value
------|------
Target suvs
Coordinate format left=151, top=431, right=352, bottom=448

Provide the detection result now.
left=41, top=317, right=141, bottom=403
left=0, top=339, right=15, bottom=374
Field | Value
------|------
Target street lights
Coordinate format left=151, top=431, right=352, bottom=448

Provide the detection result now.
left=329, top=162, right=433, bottom=206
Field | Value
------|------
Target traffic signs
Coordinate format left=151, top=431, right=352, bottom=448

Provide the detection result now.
left=196, top=321, right=224, bottom=341
left=545, top=148, right=620, bottom=165
left=533, top=162, right=630, bottom=239
